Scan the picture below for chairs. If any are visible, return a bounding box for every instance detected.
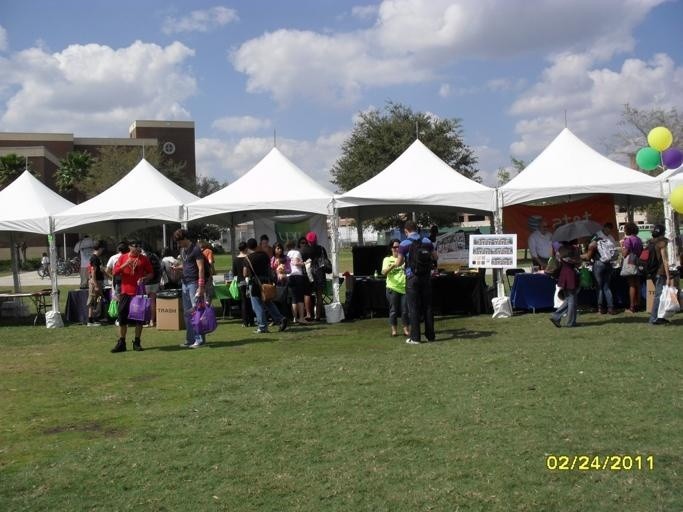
[506,269,525,292]
[39,288,61,313]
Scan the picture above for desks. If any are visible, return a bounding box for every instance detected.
[65,289,116,324]
[213,277,345,320]
[0,292,49,326]
[349,271,489,318]
[509,267,650,314]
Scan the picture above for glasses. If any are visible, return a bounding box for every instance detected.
[391,245,399,249]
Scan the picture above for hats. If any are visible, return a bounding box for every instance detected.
[306,232,317,243]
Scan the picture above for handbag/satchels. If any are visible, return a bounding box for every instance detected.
[666,282,681,311]
[318,247,332,273]
[545,256,563,276]
[246,255,276,302]
[129,283,151,322]
[657,282,675,318]
[620,238,638,276]
[192,293,216,334]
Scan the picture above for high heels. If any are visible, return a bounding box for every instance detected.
[403,328,410,336]
[391,329,397,336]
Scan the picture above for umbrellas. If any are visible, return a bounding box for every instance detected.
[549,219,603,242]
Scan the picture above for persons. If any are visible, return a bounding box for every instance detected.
[301,231,328,321]
[202,245,215,307]
[42,253,51,280]
[243,238,288,334]
[298,237,309,249]
[382,239,409,336]
[86,240,106,326]
[259,233,274,257]
[106,241,125,326]
[549,222,580,327]
[602,223,613,235]
[579,234,618,314]
[648,225,670,325]
[429,225,439,241]
[396,221,438,344]
[528,219,554,270]
[112,237,154,352]
[139,241,162,328]
[169,229,206,347]
[232,240,255,327]
[270,242,292,322]
[621,222,643,313]
[287,239,310,325]
[160,246,180,288]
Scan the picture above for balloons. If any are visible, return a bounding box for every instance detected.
[647,127,673,151]
[661,148,682,169]
[635,148,660,171]
[669,184,683,213]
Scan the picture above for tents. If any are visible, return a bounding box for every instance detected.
[496,126,661,298]
[51,159,201,310]
[184,146,339,303]
[0,170,77,310]
[657,162,683,271]
[333,139,497,302]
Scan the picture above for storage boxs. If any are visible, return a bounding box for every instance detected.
[155,298,183,331]
[646,276,679,313]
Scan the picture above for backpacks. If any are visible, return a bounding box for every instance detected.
[638,236,667,278]
[589,238,618,264]
[406,236,435,276]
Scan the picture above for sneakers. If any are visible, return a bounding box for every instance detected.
[624,307,634,313]
[406,338,421,345]
[254,326,269,333]
[132,341,143,351]
[111,340,127,352]
[189,342,206,348]
[181,343,192,347]
[278,318,287,332]
[87,322,102,327]
[549,316,562,328]
